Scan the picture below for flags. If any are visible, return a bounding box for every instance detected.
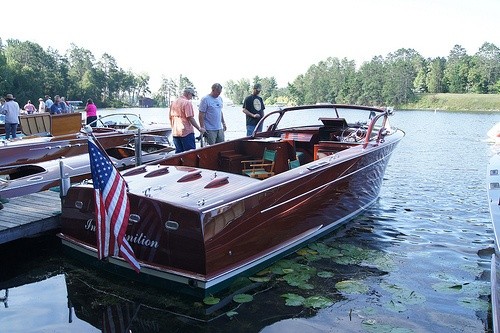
[87,134,141,274]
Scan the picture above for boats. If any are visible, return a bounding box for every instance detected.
[61,197,406,332]
[0,132,177,198]
[55,104,406,298]
[0,113,173,168]
[487,121,500,258]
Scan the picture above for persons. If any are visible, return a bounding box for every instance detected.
[84,99,98,127]
[0,98,6,113]
[38,95,75,115]
[242,83,266,137]
[24,100,37,114]
[198,83,227,146]
[4,94,21,139]
[168,87,208,155]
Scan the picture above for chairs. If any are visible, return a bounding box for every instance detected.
[288,155,300,170]
[241,146,277,178]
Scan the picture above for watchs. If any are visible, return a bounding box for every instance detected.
[222,123,226,125]
[199,128,201,130]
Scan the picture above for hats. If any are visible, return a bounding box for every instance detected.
[184,87,195,96]
[4,94,15,100]
[253,84,262,91]
